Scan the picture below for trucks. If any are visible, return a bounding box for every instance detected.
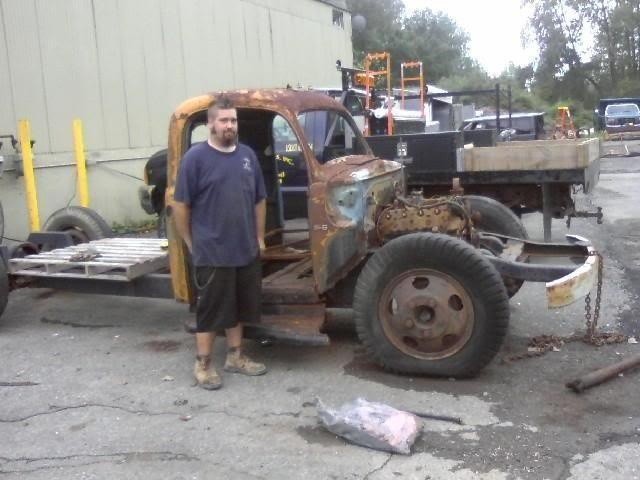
[0,86,606,380]
[594,97,640,133]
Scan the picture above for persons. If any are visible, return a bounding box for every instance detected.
[173,99,270,390]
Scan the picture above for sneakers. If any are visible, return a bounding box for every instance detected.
[194,352,222,390]
[223,350,267,377]
